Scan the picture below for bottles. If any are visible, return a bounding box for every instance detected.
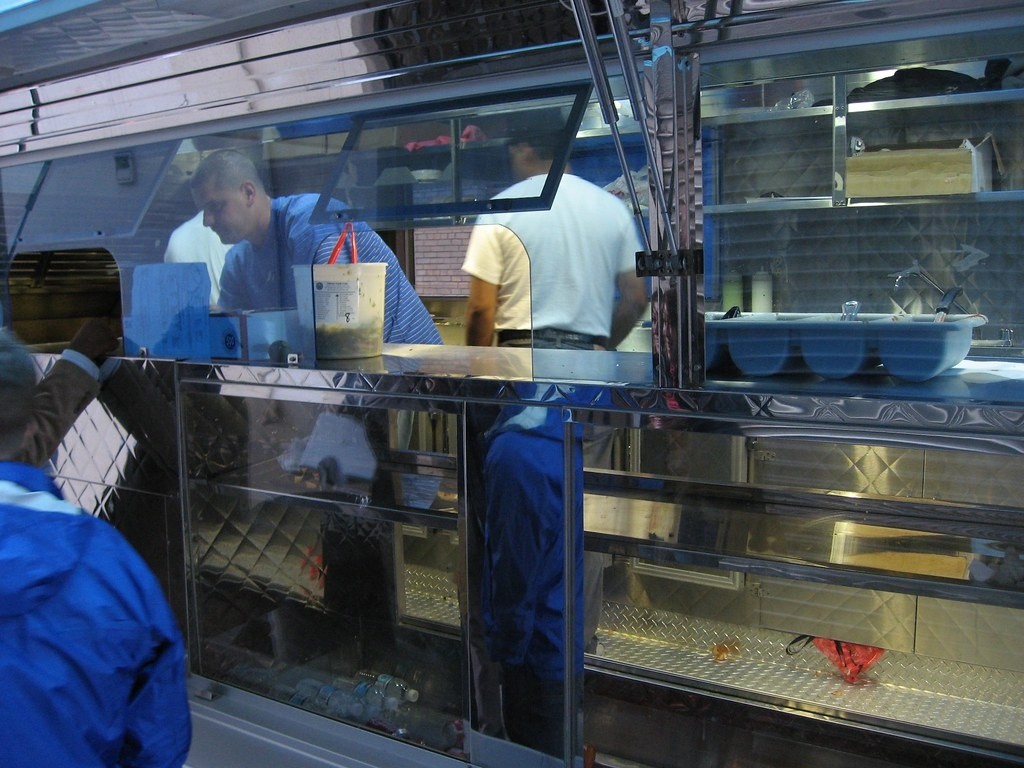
[268,654,435,719]
[722,273,743,312]
[751,266,772,313]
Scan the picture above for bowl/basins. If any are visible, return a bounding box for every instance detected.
[372,165,418,187]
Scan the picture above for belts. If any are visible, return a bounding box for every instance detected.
[498,330,610,351]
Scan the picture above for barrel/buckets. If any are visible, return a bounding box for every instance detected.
[292,222,388,360]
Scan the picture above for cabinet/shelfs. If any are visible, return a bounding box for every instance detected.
[253,28,1024,241]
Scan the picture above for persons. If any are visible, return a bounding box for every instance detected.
[0,316,191,768]
[194,148,449,508]
[479,393,584,758]
[164,208,237,311]
[460,108,651,656]
